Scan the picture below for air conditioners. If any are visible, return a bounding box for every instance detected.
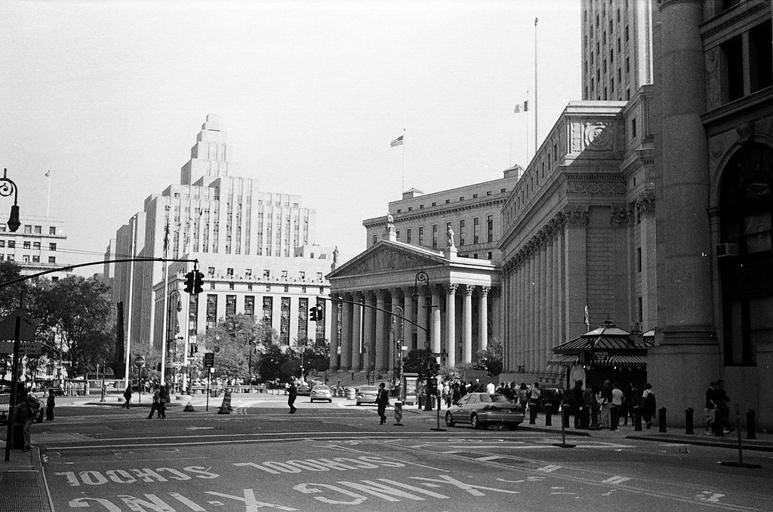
[715,242,738,257]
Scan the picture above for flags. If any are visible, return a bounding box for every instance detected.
[42,169,54,180]
[514,100,529,114]
[582,304,593,327]
[389,135,405,148]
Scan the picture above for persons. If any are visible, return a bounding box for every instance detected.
[372,374,661,428]
[145,384,165,419]
[157,385,166,418]
[716,377,735,433]
[703,381,731,441]
[122,383,135,410]
[12,385,58,453]
[286,382,298,414]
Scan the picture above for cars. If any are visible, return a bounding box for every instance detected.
[0,393,45,423]
[309,384,332,403]
[354,384,378,406]
[443,392,526,431]
[285,381,308,392]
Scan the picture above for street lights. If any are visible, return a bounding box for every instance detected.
[247,333,256,374]
[384,326,395,378]
[410,270,434,411]
[390,304,404,402]
[0,167,21,234]
[165,288,181,402]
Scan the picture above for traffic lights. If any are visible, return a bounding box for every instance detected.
[193,269,205,294]
[315,302,324,321]
[182,270,194,294]
[308,306,316,322]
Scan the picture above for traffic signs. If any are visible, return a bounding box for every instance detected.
[204,352,214,367]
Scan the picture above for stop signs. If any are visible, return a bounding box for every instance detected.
[132,356,144,368]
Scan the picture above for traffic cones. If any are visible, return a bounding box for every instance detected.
[216,389,233,415]
[182,401,195,412]
